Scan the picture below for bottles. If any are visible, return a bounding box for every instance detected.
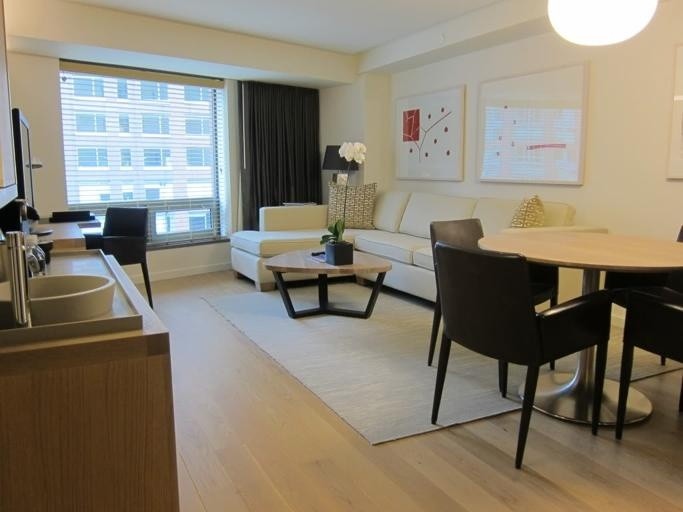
[23,233,47,277]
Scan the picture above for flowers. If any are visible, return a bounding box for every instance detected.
[319,142,368,243]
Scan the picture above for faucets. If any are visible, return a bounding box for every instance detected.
[5,230,46,328]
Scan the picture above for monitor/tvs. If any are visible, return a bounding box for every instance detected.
[11,107,33,213]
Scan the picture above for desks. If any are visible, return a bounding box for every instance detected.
[478,226,683,426]
[28,222,87,250]
[38,216,101,228]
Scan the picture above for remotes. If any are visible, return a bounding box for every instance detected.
[312,252,324,256]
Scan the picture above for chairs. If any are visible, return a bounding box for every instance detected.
[430,218,559,392]
[614,286,682,442]
[431,241,608,468]
[601,225,683,365]
[83,207,153,310]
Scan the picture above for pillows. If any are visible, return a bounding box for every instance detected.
[510,194,551,228]
[327,181,379,231]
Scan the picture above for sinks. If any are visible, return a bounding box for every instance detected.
[0,274,117,324]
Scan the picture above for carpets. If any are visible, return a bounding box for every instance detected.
[200,279,682,445]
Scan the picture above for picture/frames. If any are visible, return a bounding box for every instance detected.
[476,62,587,186]
[667,46,683,180]
[394,84,464,182]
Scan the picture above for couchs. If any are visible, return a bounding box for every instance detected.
[231,193,611,312]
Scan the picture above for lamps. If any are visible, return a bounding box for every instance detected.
[547,1,659,46]
[24,155,43,169]
[322,145,359,183]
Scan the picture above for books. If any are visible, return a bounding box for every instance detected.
[309,253,326,264]
